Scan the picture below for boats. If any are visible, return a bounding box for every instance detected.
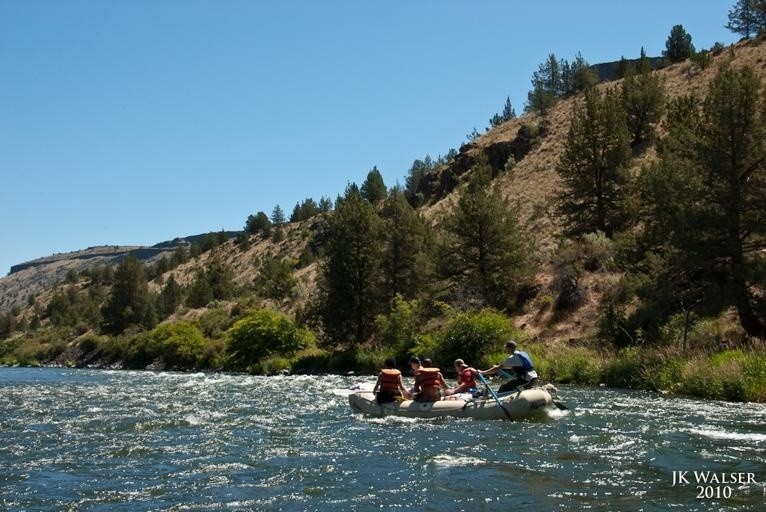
[349,387,553,418]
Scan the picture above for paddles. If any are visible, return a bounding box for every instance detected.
[478,373,513,422]
[393,395,406,400]
[493,365,570,410]
[333,389,373,396]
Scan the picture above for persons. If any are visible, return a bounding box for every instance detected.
[476,340,539,396]
[443,359,478,395]
[405,356,425,394]
[413,358,450,402]
[372,357,408,404]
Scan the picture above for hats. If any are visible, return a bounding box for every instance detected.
[454,359,468,368]
[503,340,517,348]
